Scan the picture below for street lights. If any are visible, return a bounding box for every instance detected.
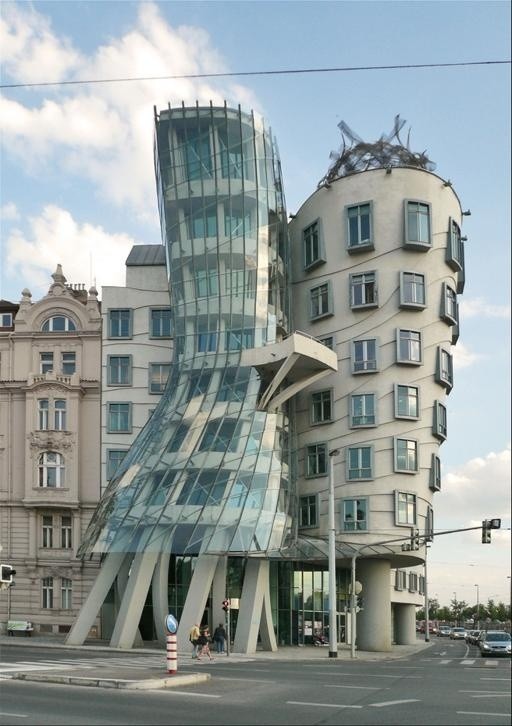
[452,590,459,627]
[326,444,344,657]
[473,583,480,630]
[506,575,512,579]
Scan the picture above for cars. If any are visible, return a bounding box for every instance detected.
[479,629,512,658]
[415,618,511,646]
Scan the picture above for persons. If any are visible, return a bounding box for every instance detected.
[189,623,201,659]
[195,625,214,661]
[211,623,227,654]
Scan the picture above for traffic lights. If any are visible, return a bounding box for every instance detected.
[355,593,362,613]
[221,599,229,612]
[1,563,17,583]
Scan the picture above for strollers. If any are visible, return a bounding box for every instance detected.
[314,634,329,646]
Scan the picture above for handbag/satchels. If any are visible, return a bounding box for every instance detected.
[196,635,207,645]
